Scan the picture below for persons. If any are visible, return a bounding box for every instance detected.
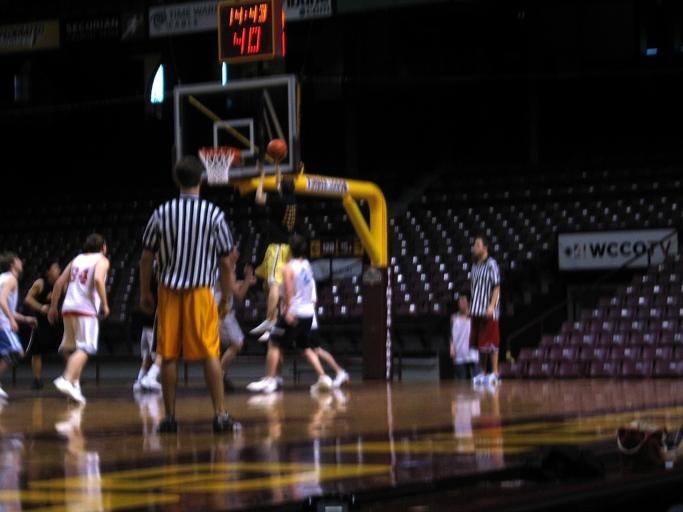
[45,232,111,398]
[136,156,235,433]
[449,292,478,380]
[248,159,297,343]
[0,249,39,402]
[131,234,351,394]
[21,258,65,391]
[469,235,501,385]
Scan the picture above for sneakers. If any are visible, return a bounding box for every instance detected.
[246,376,283,392]
[133,377,162,392]
[310,370,350,390]
[53,376,86,402]
[249,319,271,341]
[161,417,177,432]
[471,373,501,384]
[214,414,242,432]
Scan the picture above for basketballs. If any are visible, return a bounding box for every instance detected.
[267,138,288,161]
